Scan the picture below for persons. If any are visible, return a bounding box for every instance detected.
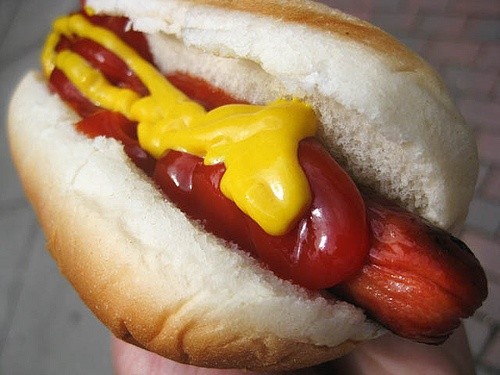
[115,317,500,375]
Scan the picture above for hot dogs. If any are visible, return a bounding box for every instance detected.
[7,1,488,371]
